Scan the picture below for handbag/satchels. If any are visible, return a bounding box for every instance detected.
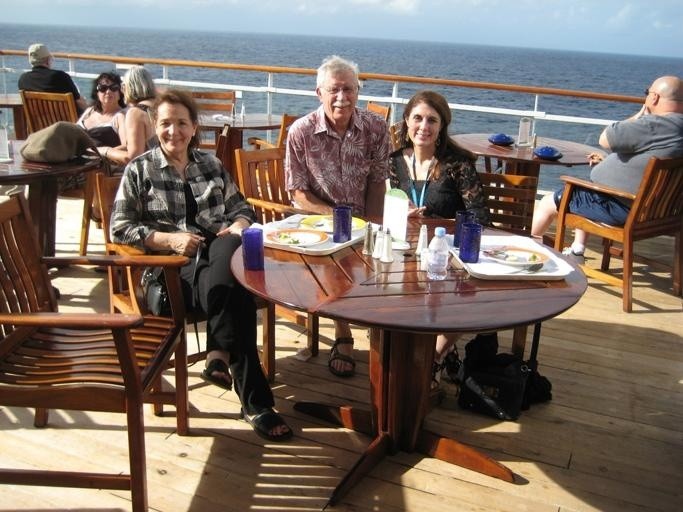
[146,280,171,316]
[20,121,96,162]
[457,364,531,422]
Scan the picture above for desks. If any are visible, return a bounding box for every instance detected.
[196,114,285,176]
[1,138,105,269]
[450,133,608,237]
[229,215,586,508]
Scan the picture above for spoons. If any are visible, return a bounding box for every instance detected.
[510,263,544,274]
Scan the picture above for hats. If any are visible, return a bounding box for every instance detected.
[29,44,51,60]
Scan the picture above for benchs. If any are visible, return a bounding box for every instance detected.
[110,91,235,148]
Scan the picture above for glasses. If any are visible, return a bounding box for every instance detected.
[643,91,657,95]
[96,83,120,92]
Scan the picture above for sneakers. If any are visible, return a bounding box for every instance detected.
[562,246,584,265]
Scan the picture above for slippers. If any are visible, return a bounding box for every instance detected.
[244,410,293,441]
[200,359,232,391]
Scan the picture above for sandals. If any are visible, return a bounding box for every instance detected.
[431,344,461,389]
[328,337,355,377]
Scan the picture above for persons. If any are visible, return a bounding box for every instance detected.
[531,75,683,265]
[284,53,390,378]
[81,66,162,166]
[18,43,88,119]
[110,86,293,441]
[57,72,127,193]
[389,91,492,397]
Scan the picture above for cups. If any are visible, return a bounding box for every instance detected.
[240,226,265,271]
[332,206,353,243]
[457,221,484,264]
[453,209,476,248]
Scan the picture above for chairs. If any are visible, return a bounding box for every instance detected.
[248,115,304,188]
[368,102,390,123]
[234,148,319,358]
[20,90,78,134]
[0,192,191,511]
[93,166,274,417]
[478,174,538,359]
[214,125,228,156]
[553,156,683,314]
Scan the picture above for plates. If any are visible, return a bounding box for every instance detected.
[301,214,367,233]
[488,133,514,145]
[266,229,330,246]
[533,146,564,160]
[479,246,553,267]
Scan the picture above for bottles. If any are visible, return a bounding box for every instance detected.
[232,120,235,127]
[231,102,236,119]
[380,228,395,263]
[424,281,446,324]
[240,101,246,118]
[371,258,383,290]
[362,220,375,256]
[414,225,431,257]
[362,254,373,280]
[241,118,245,127]
[531,132,538,150]
[420,247,430,271]
[415,256,429,290]
[379,261,392,290]
[426,225,450,280]
[372,225,384,259]
